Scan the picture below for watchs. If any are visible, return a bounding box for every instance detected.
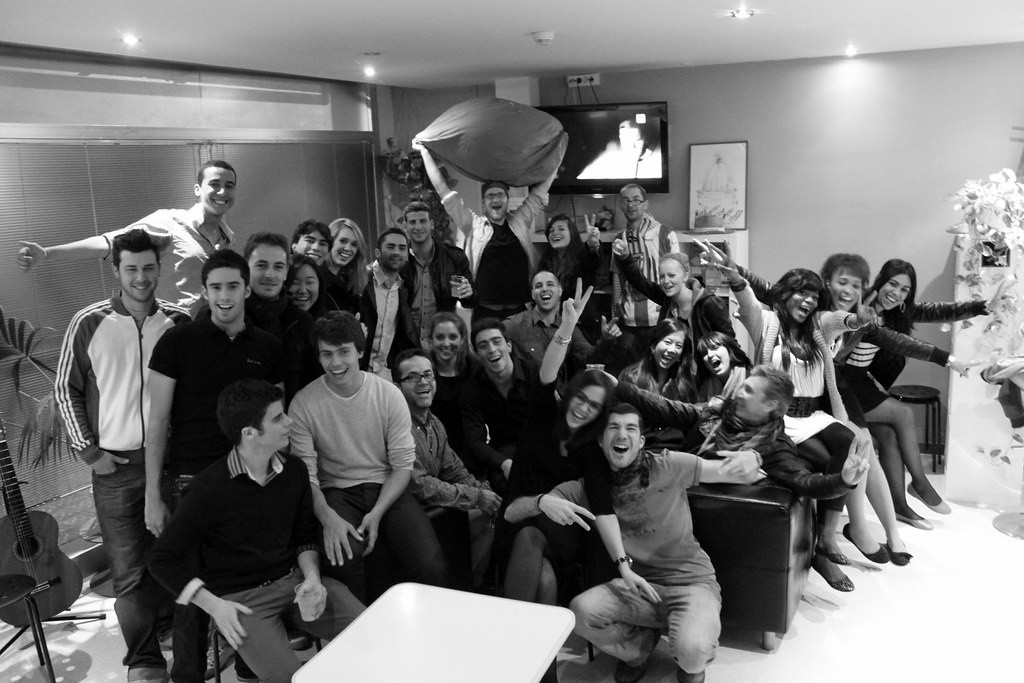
[616,555,632,567]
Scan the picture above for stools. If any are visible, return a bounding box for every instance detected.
[889,385,942,473]
[213,614,322,683]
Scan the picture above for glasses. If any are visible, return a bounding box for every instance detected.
[571,390,600,416]
[622,199,644,206]
[398,372,435,385]
[484,192,506,198]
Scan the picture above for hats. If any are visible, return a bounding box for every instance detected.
[664,252,690,272]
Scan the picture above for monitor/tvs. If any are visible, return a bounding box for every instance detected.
[533,101,670,194]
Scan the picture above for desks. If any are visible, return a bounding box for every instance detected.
[532,229,749,291]
[291,582,576,683]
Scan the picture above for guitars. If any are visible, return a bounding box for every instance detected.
[0,418,85,627]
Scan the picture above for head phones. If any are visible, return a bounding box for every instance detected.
[721,399,750,433]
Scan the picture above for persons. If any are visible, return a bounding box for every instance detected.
[16,132,1015,683]
[619,121,651,161]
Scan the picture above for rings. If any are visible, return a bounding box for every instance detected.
[493,508,497,511]
[146,527,150,529]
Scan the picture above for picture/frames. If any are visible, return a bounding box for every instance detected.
[687,140,749,230]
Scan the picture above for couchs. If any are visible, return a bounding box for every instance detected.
[686,451,824,650]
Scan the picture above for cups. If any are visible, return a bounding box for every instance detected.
[586,364,605,372]
[294,583,321,621]
[452,275,463,298]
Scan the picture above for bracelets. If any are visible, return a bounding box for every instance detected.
[587,241,600,257]
[946,355,956,366]
[537,495,544,508]
[554,329,571,345]
[721,277,742,284]
[847,315,860,331]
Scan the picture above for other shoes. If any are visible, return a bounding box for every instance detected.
[811,552,856,592]
[678,664,705,683]
[290,637,310,651]
[235,667,258,681]
[843,524,891,564]
[203,644,234,680]
[895,507,932,530]
[616,628,662,683]
[815,542,850,565]
[886,539,911,566]
[128,665,170,683]
[908,481,951,514]
